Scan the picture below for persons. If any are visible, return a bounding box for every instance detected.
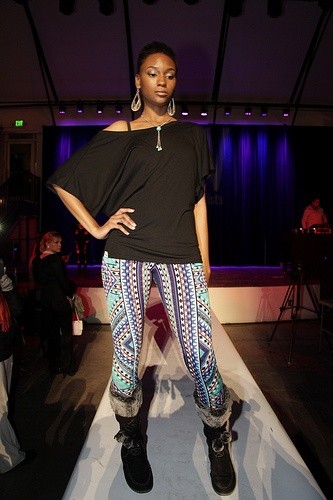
[0,231,78,472]
[300,196,330,229]
[47,41,244,496]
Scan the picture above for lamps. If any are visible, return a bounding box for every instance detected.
[182,102,290,118]
[58,0,333,18]
[58,103,121,114]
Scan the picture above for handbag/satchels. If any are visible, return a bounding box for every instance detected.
[72,306,83,336]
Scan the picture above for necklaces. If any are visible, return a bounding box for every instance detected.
[142,115,173,151]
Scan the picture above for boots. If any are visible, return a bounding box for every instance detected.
[192,384,237,495]
[107,383,155,494]
